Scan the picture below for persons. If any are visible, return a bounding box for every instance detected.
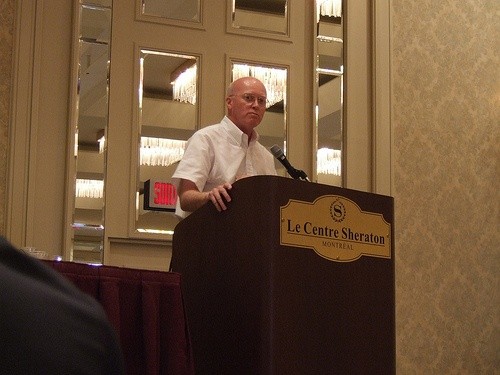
[0,234,123,375]
[174,77,279,222]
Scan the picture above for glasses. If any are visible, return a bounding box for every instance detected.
[228,94,269,104]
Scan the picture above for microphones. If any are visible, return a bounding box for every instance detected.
[270,144,300,181]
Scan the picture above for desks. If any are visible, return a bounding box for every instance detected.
[38,259,196,375]
